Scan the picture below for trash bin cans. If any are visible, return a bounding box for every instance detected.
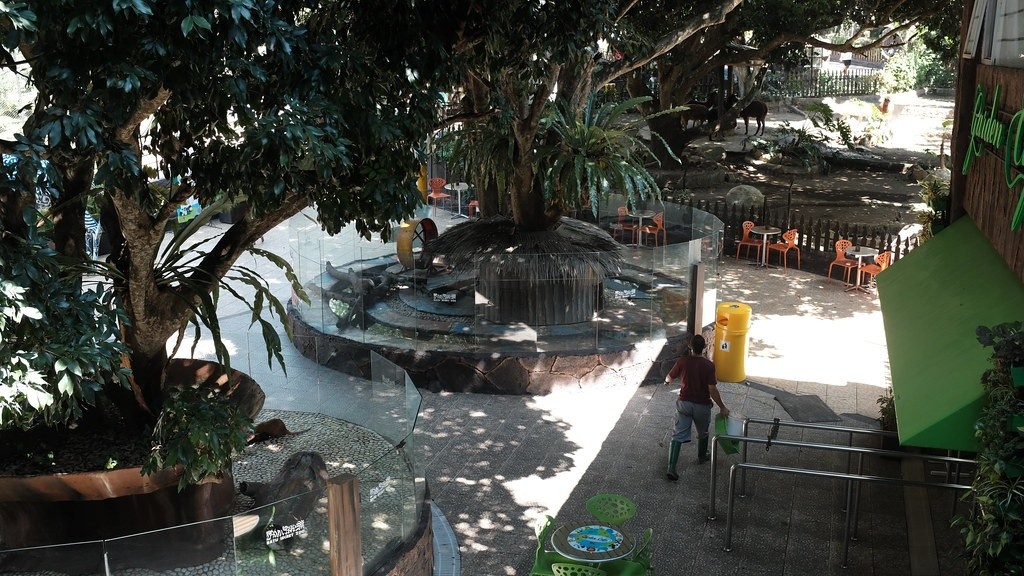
[715,301,752,384]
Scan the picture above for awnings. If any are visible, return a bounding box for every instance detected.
[875,214,1024,451]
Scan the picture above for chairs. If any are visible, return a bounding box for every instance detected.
[426,178,453,217]
[766,229,800,274]
[828,240,866,288]
[641,211,668,250]
[613,207,639,244]
[469,201,479,217]
[736,221,770,267]
[528,493,653,576]
[856,251,892,299]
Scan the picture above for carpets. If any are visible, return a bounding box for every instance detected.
[776,394,843,423]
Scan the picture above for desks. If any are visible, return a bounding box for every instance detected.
[551,520,637,568]
[844,246,880,294]
[444,182,474,219]
[626,210,656,249]
[746,226,781,270]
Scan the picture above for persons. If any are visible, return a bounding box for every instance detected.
[840,52,852,73]
[665,334,729,480]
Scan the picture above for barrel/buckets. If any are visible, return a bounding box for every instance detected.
[727,411,746,436]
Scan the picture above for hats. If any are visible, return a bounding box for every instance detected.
[687,334,708,348]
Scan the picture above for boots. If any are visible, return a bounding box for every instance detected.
[697,433,711,463]
[666,440,682,481]
